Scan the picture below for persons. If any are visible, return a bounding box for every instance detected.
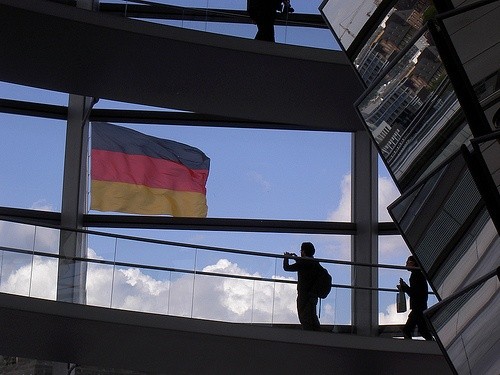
[282,241,332,328]
[397,255,435,341]
[247,0,295,43]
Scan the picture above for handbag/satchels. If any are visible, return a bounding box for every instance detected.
[396,280,407,313]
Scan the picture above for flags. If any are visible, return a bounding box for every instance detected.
[90,121,210,216]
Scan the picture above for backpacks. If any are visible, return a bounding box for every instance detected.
[312,264,332,299]
